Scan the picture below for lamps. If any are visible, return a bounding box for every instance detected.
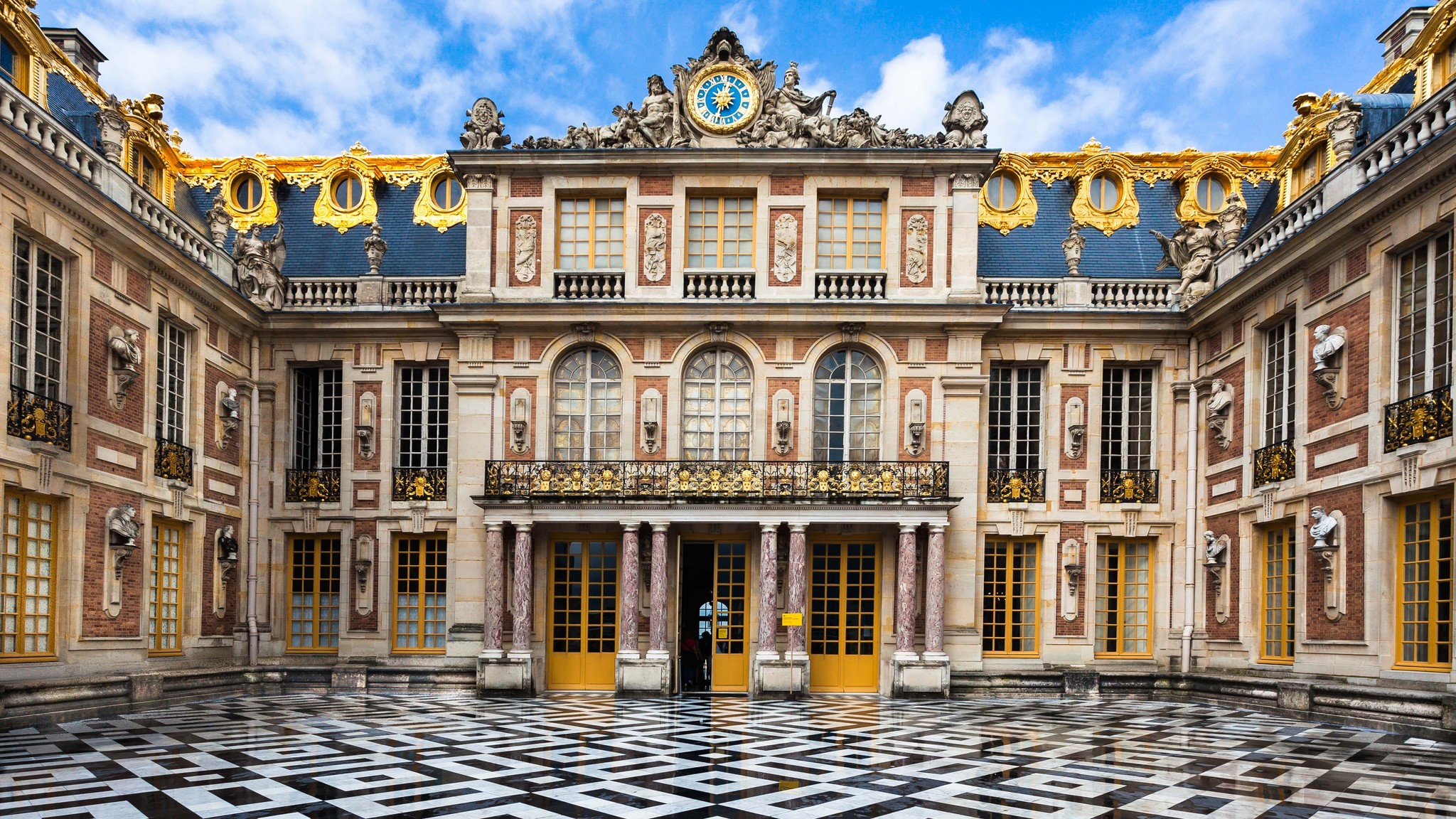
[509,389,534,454]
[640,391,661,455]
[1063,542,1083,598]
[905,394,926,457]
[1065,401,1085,459]
[770,392,795,456]
[353,535,373,595]
[354,393,378,459]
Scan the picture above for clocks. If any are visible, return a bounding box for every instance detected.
[684,64,763,134]
